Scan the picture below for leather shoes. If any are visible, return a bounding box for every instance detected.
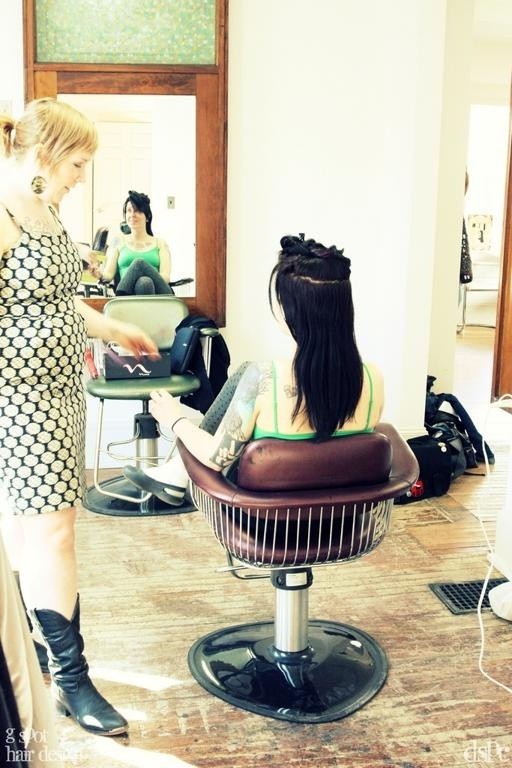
[122,464,187,507]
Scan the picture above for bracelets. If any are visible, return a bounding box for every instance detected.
[171,416,188,431]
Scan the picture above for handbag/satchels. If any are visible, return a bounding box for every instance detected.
[393,435,452,504]
[428,423,467,480]
[426,372,494,467]
[170,326,199,375]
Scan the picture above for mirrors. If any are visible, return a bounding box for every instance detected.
[22,66,230,334]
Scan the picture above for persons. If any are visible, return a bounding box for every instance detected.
[4,96,161,740]
[88,188,175,297]
[124,230,387,550]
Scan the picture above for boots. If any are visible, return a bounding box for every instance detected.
[12,571,49,672]
[25,593,129,737]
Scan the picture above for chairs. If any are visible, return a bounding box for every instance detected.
[81,296,423,725]
